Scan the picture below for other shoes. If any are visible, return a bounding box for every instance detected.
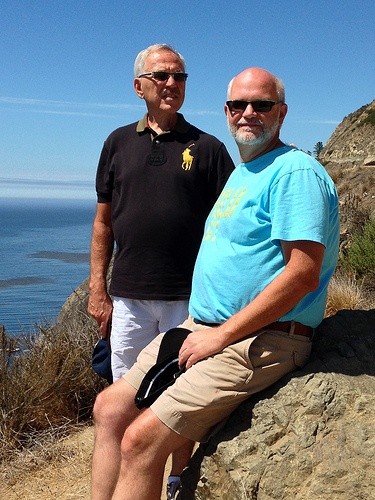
[166,475,181,500]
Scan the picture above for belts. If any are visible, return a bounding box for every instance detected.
[193,317,313,340]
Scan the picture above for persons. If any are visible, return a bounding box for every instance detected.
[91,66,341,500]
[86,43,236,500]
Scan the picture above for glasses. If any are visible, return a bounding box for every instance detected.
[138,72,188,82]
[226,100,283,112]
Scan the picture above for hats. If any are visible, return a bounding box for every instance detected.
[91,319,113,385]
[134,328,196,409]
